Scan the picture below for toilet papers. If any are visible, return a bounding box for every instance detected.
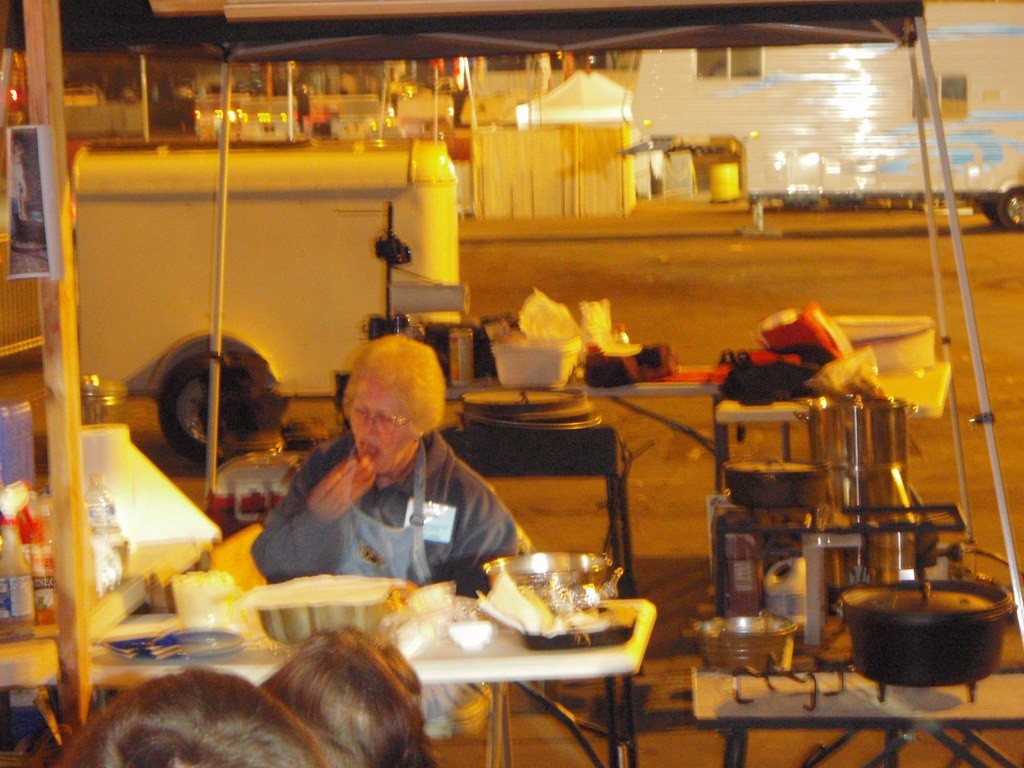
[81,423,223,585]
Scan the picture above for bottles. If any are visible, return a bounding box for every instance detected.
[0,519,36,642]
[723,510,757,619]
[763,558,807,625]
[615,321,630,342]
[30,506,54,625]
[84,474,116,536]
[450,327,475,387]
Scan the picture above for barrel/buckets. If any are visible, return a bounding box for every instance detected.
[713,163,738,198]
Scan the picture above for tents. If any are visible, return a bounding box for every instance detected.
[0,0,1024,732]
[515,69,633,125]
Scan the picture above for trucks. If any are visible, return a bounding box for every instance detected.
[2,138,464,468]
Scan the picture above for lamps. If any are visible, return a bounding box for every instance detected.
[514,51,632,130]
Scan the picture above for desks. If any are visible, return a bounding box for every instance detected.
[691,666,1024,768]
[444,366,953,616]
[0,598,658,768]
[0,538,212,753]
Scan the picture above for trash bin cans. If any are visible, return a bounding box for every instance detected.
[710,162,740,202]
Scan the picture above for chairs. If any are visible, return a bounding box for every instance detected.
[443,409,639,768]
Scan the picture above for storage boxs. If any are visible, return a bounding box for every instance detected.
[834,312,936,373]
[490,339,578,388]
[472,125,637,222]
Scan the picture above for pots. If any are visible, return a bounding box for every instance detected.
[722,456,832,506]
[840,579,1014,687]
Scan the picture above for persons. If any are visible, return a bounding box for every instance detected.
[260,628,436,768]
[250,334,518,586]
[52,667,327,768]
[11,139,28,236]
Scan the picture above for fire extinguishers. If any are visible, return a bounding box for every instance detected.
[705,490,762,618]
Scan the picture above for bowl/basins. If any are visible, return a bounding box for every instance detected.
[483,551,614,614]
[698,612,799,673]
[450,621,490,650]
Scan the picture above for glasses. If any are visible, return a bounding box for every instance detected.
[344,404,410,433]
